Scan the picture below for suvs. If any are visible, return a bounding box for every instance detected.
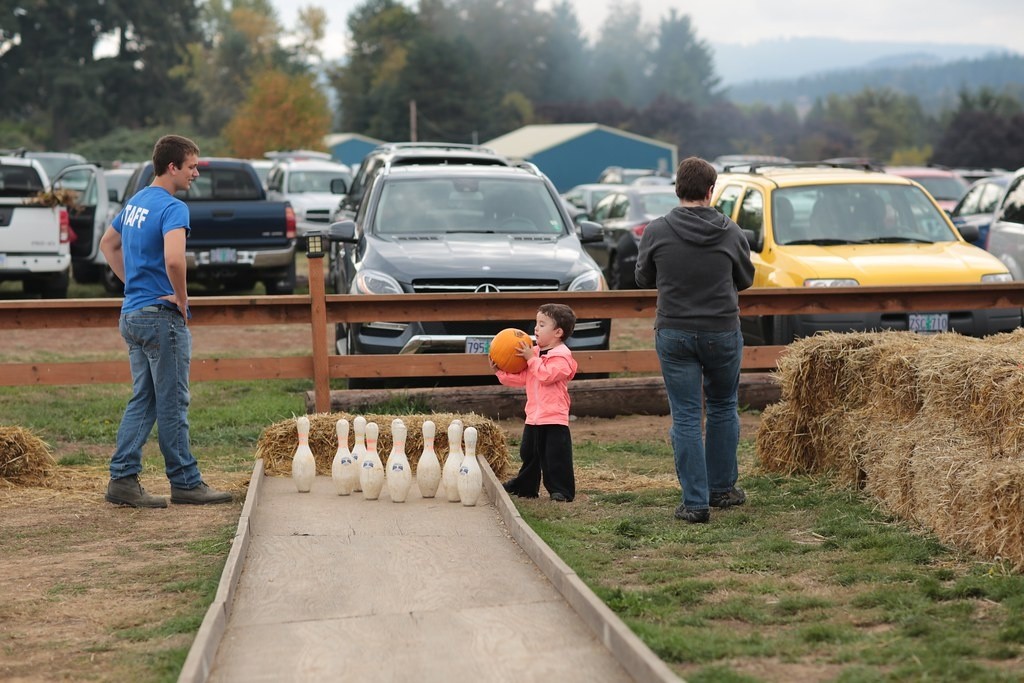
[326,161,611,390]
[329,141,508,289]
[709,162,1022,344]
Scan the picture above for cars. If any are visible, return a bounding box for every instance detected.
[599,167,660,189]
[1,151,91,190]
[263,160,354,237]
[74,170,136,284]
[986,167,1024,283]
[559,185,630,229]
[710,165,817,229]
[877,167,971,236]
[932,177,1011,252]
[577,189,680,290]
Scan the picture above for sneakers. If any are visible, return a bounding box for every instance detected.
[170,480,233,504]
[105,473,167,508]
[674,504,710,523]
[709,488,746,508]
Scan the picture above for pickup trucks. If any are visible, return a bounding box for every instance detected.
[102,157,296,297]
[0,157,111,301]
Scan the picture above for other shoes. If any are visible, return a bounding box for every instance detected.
[551,493,568,501]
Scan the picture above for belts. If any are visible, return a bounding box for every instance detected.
[151,304,173,312]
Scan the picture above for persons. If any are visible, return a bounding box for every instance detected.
[635,157,755,522]
[100,136,232,508]
[488,304,578,503]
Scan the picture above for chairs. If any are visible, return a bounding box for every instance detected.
[484,191,530,230]
[774,195,809,241]
[388,192,440,228]
[809,193,885,238]
